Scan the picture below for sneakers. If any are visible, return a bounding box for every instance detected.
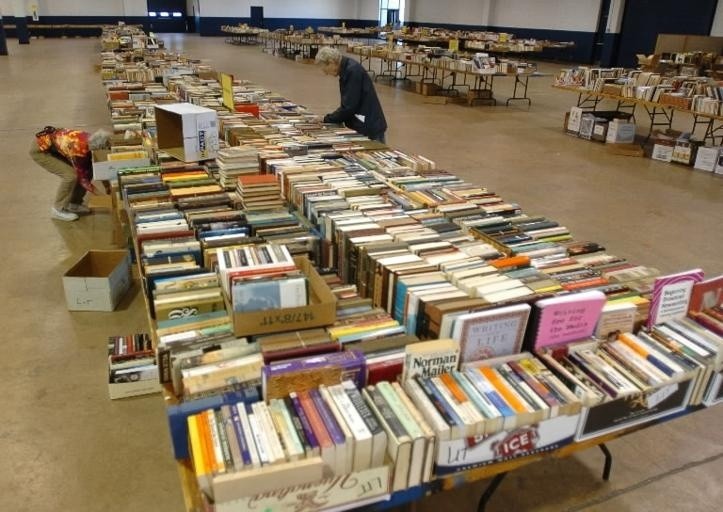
[49,206,79,222]
[66,202,90,213]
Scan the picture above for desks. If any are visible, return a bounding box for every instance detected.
[128,216,704,512]
[225,32,259,44]
[261,36,347,60]
[346,51,533,106]
[551,84,723,149]
[323,31,371,45]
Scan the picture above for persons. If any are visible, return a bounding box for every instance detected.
[28,127,113,221]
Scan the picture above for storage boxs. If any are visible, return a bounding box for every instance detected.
[416,81,437,96]
[62,250,133,312]
[154,103,219,163]
[468,89,490,105]
[108,357,162,400]
[92,149,150,181]
[564,107,723,175]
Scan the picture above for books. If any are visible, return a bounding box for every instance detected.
[98,21,722,512]
